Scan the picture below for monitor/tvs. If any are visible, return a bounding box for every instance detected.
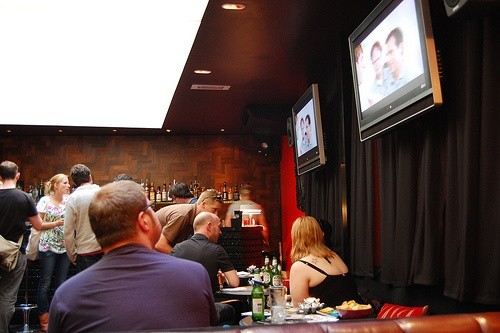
[348,0,444,142]
[291,83,326,176]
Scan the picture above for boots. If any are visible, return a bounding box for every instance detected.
[39,313,49,331]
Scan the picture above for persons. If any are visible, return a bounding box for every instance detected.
[169,182,198,205]
[227,181,271,253]
[153,187,224,258]
[48,180,221,333]
[291,112,316,157]
[63,163,104,273]
[315,219,344,264]
[354,27,422,109]
[35,173,73,333]
[0,161,65,333]
[171,210,240,327]
[288,216,362,308]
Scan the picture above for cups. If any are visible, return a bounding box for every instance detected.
[217,272,224,290]
[269,286,287,325]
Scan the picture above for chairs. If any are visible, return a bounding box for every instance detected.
[376,303,429,320]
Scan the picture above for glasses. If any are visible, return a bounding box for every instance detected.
[202,195,223,202]
[141,201,156,213]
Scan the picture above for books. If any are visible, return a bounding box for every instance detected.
[221,287,247,292]
[241,306,340,325]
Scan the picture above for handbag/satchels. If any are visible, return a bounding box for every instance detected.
[0,235,22,269]
[26,197,47,260]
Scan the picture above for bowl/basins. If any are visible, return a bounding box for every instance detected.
[336,304,373,319]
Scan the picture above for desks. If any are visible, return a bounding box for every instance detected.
[217,271,265,301]
[239,317,374,327]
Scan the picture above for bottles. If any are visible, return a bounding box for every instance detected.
[71,184,77,193]
[24,322,29,333]
[251,255,283,322]
[16,180,45,201]
[140,177,240,202]
